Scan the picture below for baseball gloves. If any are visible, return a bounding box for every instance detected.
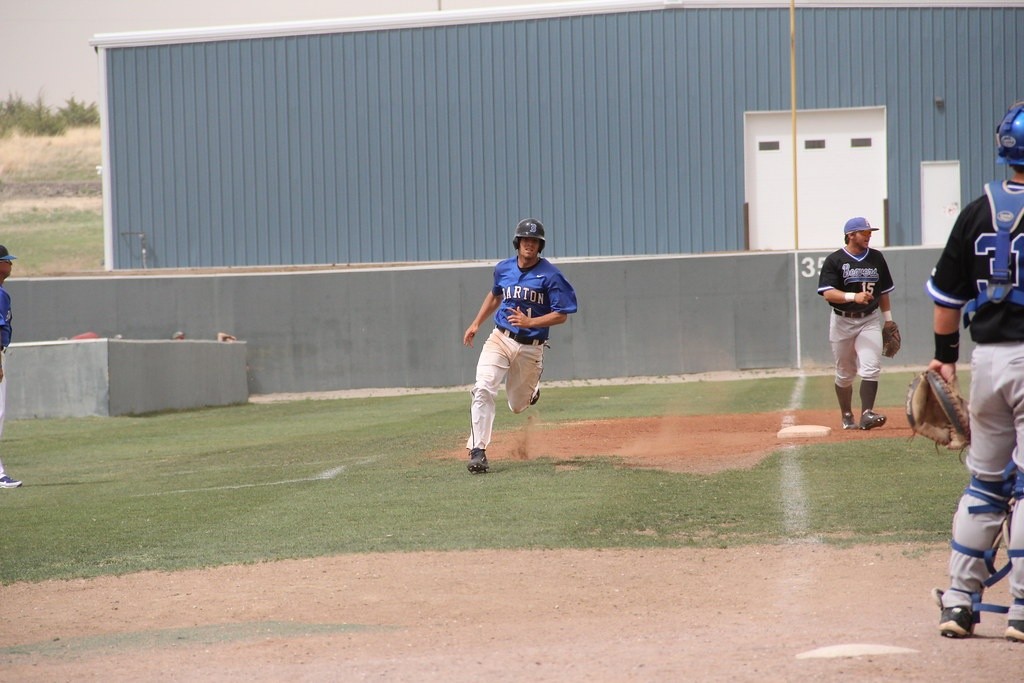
[882,320,902,358]
[905,371,973,453]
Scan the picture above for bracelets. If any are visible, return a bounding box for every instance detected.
[934,329,960,363]
[0,365,2,368]
[845,293,856,301]
[883,311,892,321]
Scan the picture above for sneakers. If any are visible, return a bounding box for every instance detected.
[1004,619,1024,642]
[467,448,490,473]
[841,412,858,429]
[858,410,887,429]
[939,605,977,638]
[531,388,540,405]
[1,474,23,489]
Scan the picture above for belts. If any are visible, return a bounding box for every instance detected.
[497,324,546,346]
[833,304,880,318]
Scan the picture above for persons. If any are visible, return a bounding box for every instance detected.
[818,218,902,430]
[464,219,578,472]
[0,244,23,488]
[925,101,1024,641]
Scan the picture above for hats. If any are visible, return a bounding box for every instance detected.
[0,243,17,260]
[844,217,880,233]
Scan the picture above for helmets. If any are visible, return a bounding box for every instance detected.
[514,218,545,253]
[996,102,1024,166]
